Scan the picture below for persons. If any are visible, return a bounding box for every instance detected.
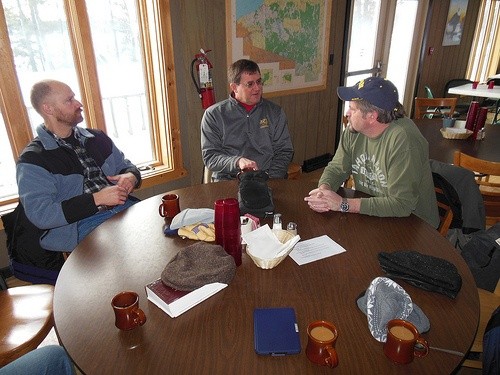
[303,76,440,231]
[200,59,295,183]
[0,345,77,375]
[15,80,142,253]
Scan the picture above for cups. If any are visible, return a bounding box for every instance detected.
[159,193,181,220]
[215,198,242,267]
[383,320,429,364]
[443,117,456,128]
[111,291,146,331]
[488,81,494,89]
[240,215,257,244]
[454,120,466,129]
[465,100,488,140]
[472,80,479,89]
[305,321,339,369]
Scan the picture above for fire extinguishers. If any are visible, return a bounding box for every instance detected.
[196,48,216,109]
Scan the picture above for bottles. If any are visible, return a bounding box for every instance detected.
[272,214,283,229]
[287,221,297,235]
[263,211,274,229]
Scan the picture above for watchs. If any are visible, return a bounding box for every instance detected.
[341,198,349,214]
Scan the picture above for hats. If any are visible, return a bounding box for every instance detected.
[336,76,398,112]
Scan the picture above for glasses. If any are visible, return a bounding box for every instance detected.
[236,79,263,87]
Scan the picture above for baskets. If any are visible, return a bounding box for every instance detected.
[245,228,296,270]
[440,126,474,140]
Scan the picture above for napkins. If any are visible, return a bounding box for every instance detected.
[243,223,301,259]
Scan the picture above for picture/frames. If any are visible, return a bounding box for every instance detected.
[442,0,469,46]
[225,0,332,99]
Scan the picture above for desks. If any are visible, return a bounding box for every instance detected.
[413,120,500,165]
[53,179,480,374]
[448,83,500,99]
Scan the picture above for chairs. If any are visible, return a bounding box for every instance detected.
[0,198,69,368]
[341,78,500,375]
